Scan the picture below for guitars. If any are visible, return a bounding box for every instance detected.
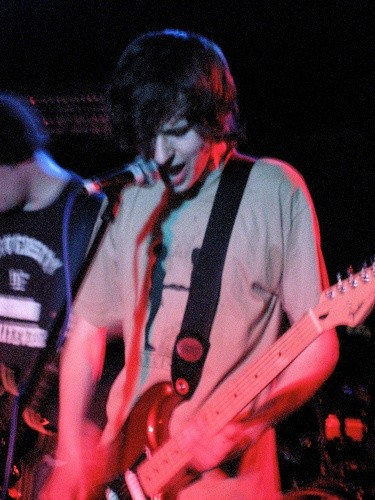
[35,262,375,500]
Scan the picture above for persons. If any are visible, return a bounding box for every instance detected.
[34,27,340,500]
[0,92,128,500]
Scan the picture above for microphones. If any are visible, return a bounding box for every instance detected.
[75,158,159,197]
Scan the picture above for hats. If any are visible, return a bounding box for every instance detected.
[0,93,47,165]
